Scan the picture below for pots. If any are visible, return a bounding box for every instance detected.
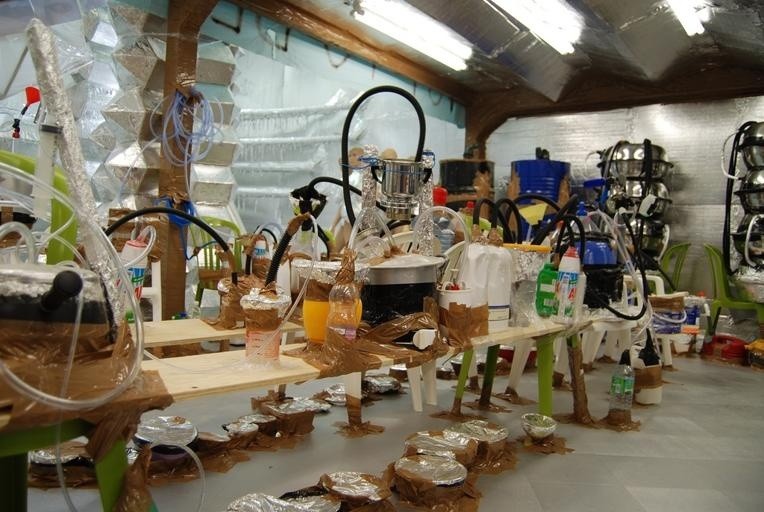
[0,264,109,325]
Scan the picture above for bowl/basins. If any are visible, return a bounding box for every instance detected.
[521,412,557,440]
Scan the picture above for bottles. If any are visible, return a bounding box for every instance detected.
[117,239,149,325]
[606,348,636,425]
[454,200,473,243]
[251,240,267,275]
[551,244,580,326]
[323,280,359,346]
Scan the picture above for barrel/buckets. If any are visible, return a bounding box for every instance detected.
[439,157,495,213]
[511,158,570,242]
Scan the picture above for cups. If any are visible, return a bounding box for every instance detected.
[238,293,292,369]
